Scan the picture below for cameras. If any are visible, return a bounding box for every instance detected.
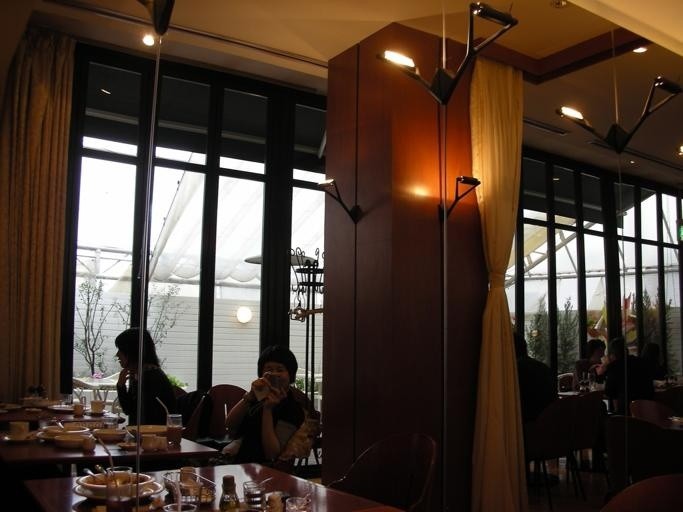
[270,372,289,388]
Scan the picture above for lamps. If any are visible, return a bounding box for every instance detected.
[373,1,523,108]
[556,77,683,150]
[316,177,365,226]
[438,174,482,218]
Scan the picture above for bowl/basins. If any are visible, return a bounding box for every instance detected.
[126,425,186,438]
[93,429,125,440]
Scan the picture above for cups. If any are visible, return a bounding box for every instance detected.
[242,481,312,512]
[89,400,105,413]
[73,403,86,416]
[104,472,132,512]
[140,433,166,451]
[82,436,96,451]
[9,422,29,439]
[162,502,197,512]
[101,418,118,430]
[180,467,196,484]
[167,414,182,446]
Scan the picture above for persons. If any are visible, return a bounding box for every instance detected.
[114,326,176,424]
[513,331,559,423]
[588,336,657,471]
[221,344,305,465]
[573,339,608,417]
[639,343,666,378]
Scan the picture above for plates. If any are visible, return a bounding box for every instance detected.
[1,435,35,443]
[47,404,90,412]
[74,471,164,503]
[117,443,137,450]
[70,498,155,512]
[34,425,92,447]
[86,410,110,415]
[25,408,42,413]
[1,404,21,410]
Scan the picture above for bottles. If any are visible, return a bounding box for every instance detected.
[218,474,241,512]
[579,372,598,393]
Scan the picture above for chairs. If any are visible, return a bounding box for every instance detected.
[331,432,441,511]
[516,380,682,510]
[186,384,248,454]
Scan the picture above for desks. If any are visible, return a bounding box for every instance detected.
[1,407,405,511]
